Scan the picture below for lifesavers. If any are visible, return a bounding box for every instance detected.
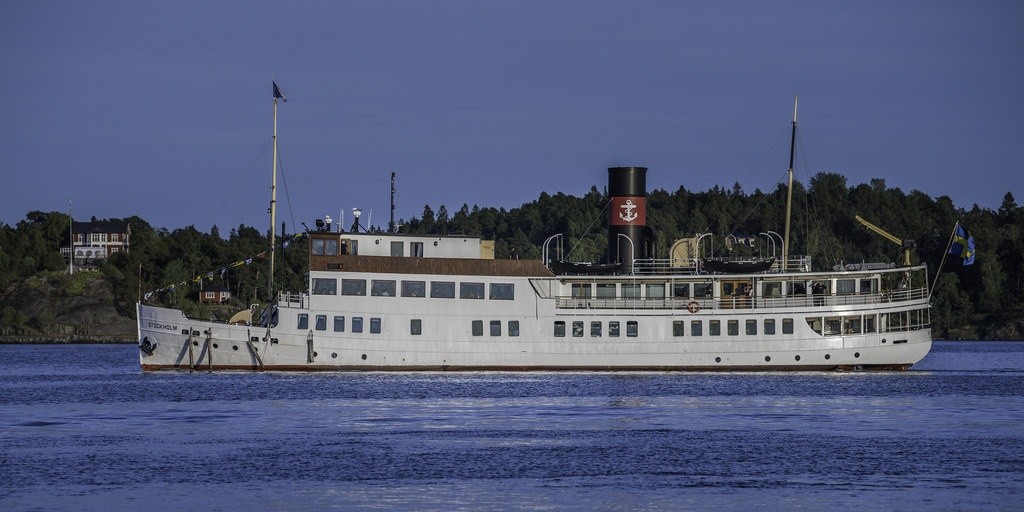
[687,301,700,314]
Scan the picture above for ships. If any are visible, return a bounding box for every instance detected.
[136,79,977,378]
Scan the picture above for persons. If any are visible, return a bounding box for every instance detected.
[812,282,828,305]
[897,275,908,301]
[317,286,496,299]
[705,284,752,308]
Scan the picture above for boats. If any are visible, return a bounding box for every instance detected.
[703,255,775,273]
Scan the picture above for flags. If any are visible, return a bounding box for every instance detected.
[272,80,288,103]
[949,223,974,265]
